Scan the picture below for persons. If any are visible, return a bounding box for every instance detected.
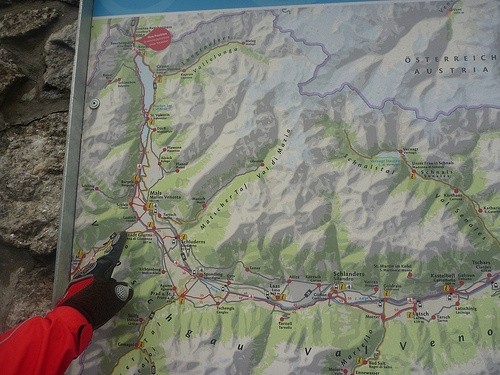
[0,230,134,375]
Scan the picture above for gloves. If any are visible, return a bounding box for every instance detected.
[53,229,134,329]
